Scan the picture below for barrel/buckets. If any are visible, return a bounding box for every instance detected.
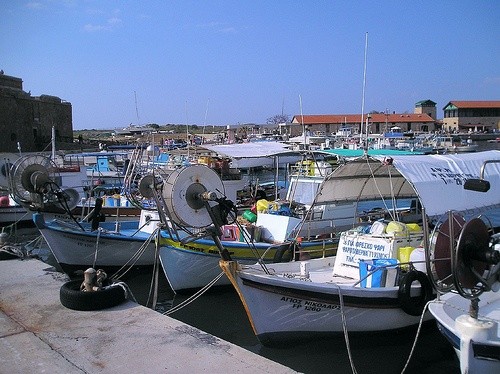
[244,226,261,243]
[241,210,257,222]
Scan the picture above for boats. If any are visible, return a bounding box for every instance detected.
[0,32,500,374]
